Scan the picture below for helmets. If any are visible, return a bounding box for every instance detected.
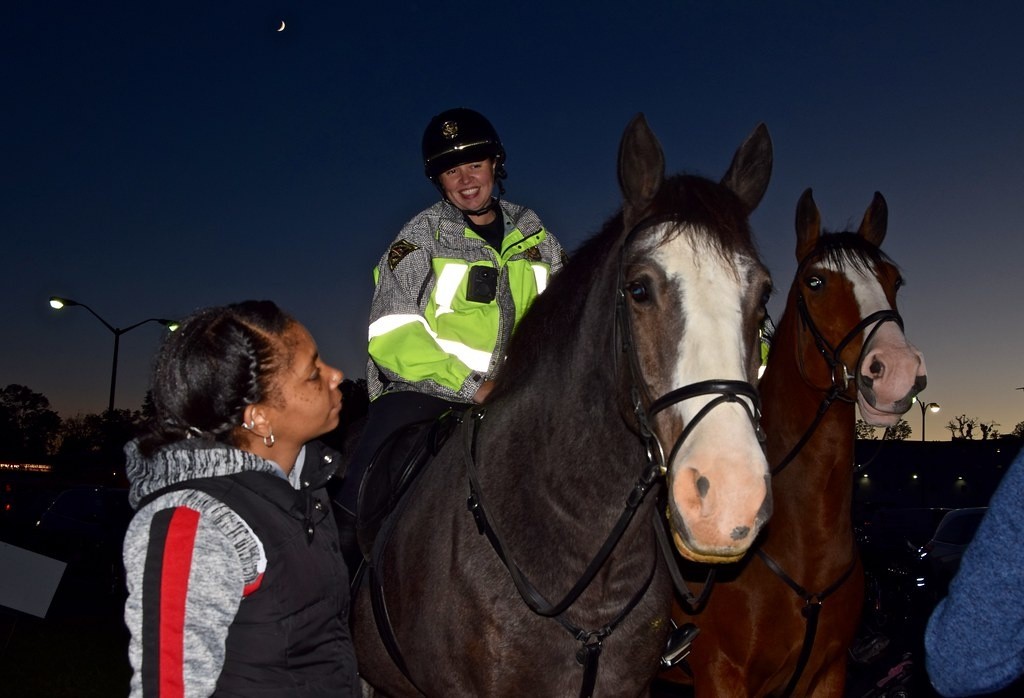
[422,108,506,182]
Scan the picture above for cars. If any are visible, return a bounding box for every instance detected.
[915,506,988,574]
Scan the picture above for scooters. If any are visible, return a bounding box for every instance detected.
[843,630,916,698]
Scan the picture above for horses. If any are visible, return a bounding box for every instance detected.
[328,114,777,698]
[655,187,928,698]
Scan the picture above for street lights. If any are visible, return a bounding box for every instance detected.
[912,394,939,440]
[49,295,180,422]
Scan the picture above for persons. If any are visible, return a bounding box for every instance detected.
[122,299,363,698]
[755,266,777,381]
[923,450,1024,697]
[336,108,564,564]
